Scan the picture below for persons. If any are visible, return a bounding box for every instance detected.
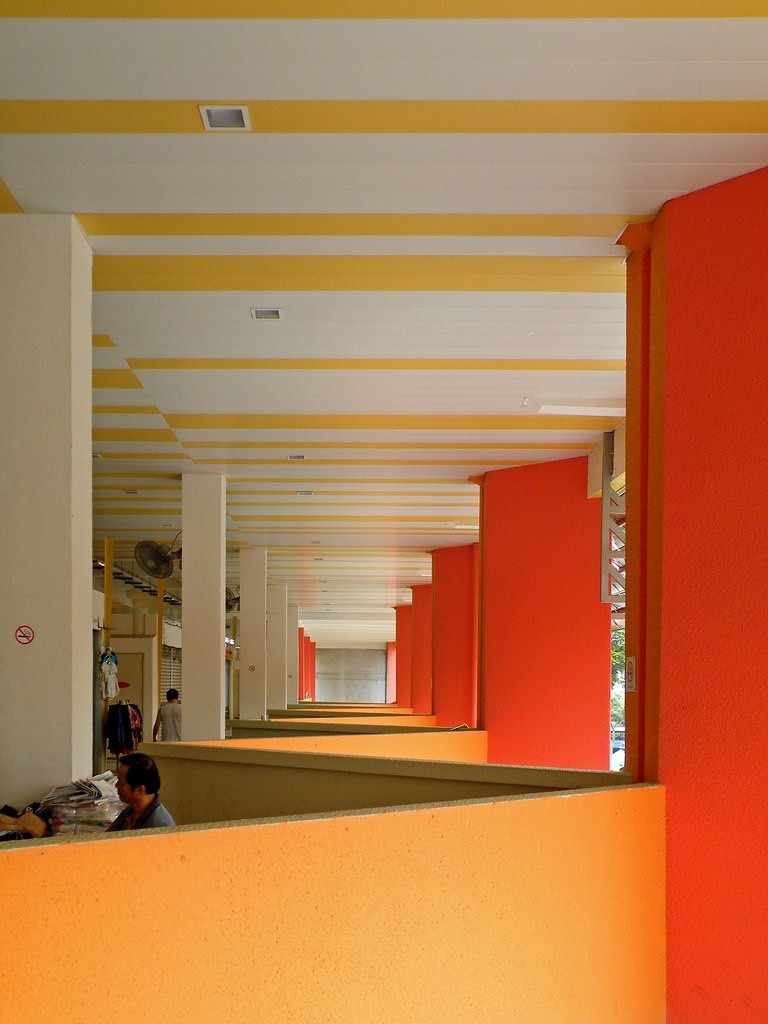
[102,752,177,832]
[152,687,183,743]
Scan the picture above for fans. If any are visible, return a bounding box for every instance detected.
[135,540,182,580]
[225,585,240,613]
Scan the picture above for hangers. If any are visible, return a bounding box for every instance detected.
[97,642,132,711]
